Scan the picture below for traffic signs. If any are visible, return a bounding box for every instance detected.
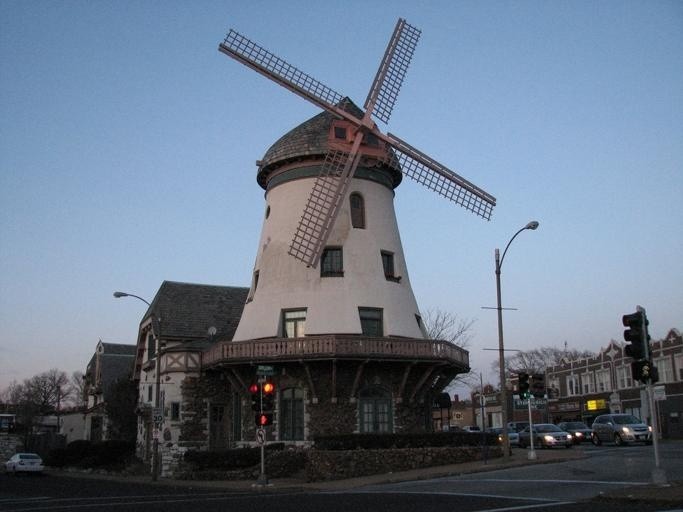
[152,408,164,423]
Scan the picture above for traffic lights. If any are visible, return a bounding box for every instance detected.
[532,374,546,400]
[263,381,275,411]
[255,414,274,427]
[629,361,653,383]
[621,311,647,362]
[250,380,262,413]
[518,371,531,402]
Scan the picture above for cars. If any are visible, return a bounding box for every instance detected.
[440,410,656,450]
[4,453,46,478]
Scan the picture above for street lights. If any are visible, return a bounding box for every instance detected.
[492,219,541,465]
[111,290,166,489]
